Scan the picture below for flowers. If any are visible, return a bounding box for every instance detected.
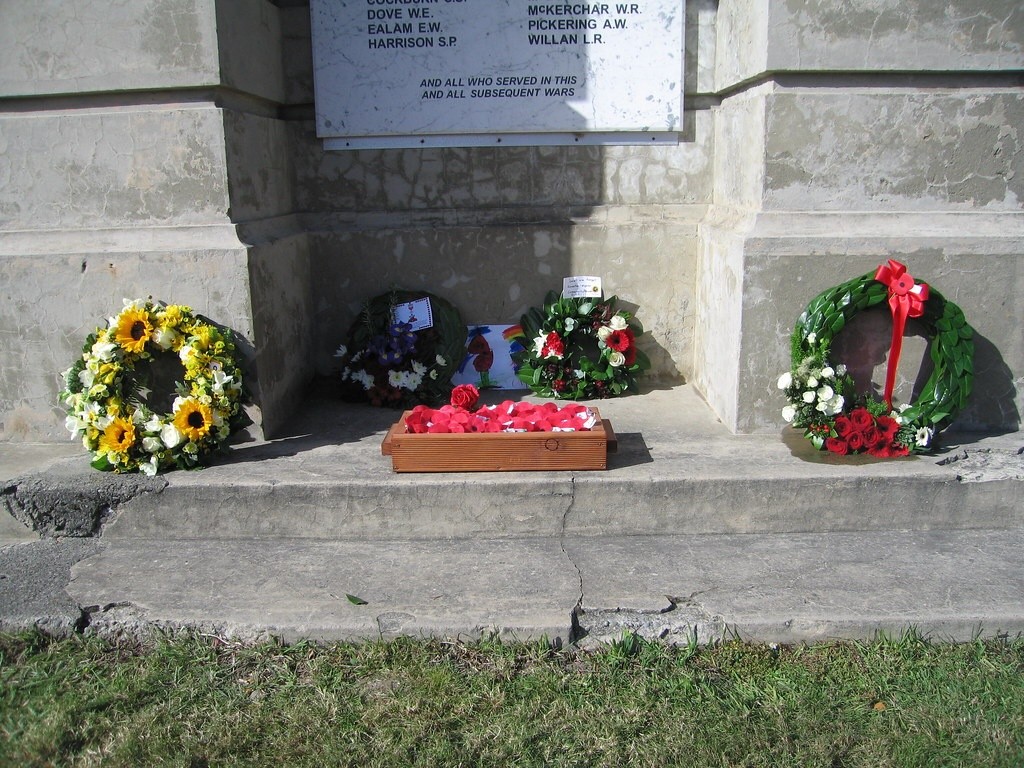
[332,288,467,410]
[404,400,596,433]
[509,285,652,402]
[55,295,253,479]
[449,383,480,411]
[778,264,976,459]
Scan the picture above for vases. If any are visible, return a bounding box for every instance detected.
[381,410,617,472]
